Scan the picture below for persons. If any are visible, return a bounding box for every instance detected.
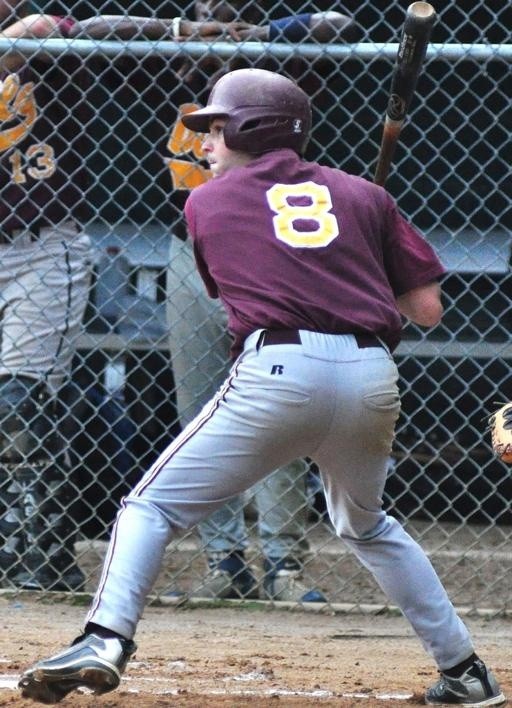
[0,0,98,590]
[20,66,508,708]
[64,0,362,603]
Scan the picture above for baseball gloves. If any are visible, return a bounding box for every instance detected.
[480,400,511,463]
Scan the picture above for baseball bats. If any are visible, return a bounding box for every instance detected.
[374,1,436,184]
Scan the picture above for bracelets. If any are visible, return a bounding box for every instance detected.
[266,12,312,44]
[172,16,182,39]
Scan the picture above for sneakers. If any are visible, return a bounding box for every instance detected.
[17,631,137,703]
[425,658,507,707]
[263,555,327,601]
[165,548,259,598]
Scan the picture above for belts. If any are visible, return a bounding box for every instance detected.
[0,226,39,244]
[258,328,383,348]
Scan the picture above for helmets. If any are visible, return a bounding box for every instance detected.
[181,67,313,158]
